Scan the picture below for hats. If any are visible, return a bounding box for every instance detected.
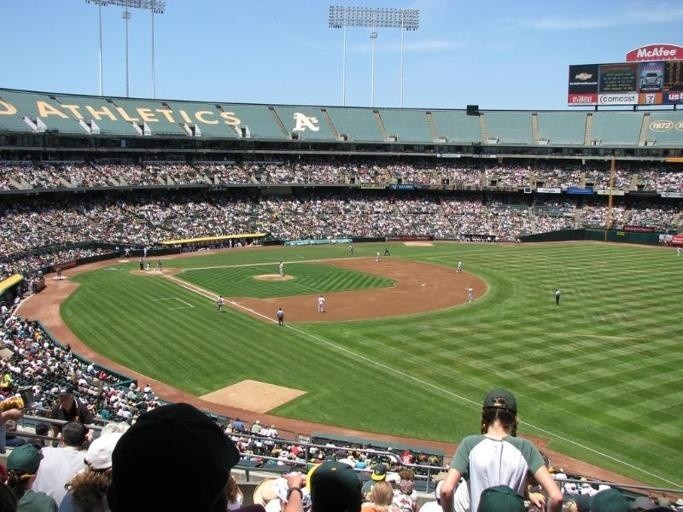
[483,388,517,411]
[477,485,524,511]
[371,465,386,480]
[590,488,630,511]
[112,404,240,511]
[85,433,126,470]
[252,475,280,506]
[305,461,363,503]
[7,444,44,475]
[59,389,72,396]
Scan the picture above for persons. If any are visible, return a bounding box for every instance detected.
[1,152,682,511]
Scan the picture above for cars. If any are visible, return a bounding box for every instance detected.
[640,73,662,87]
